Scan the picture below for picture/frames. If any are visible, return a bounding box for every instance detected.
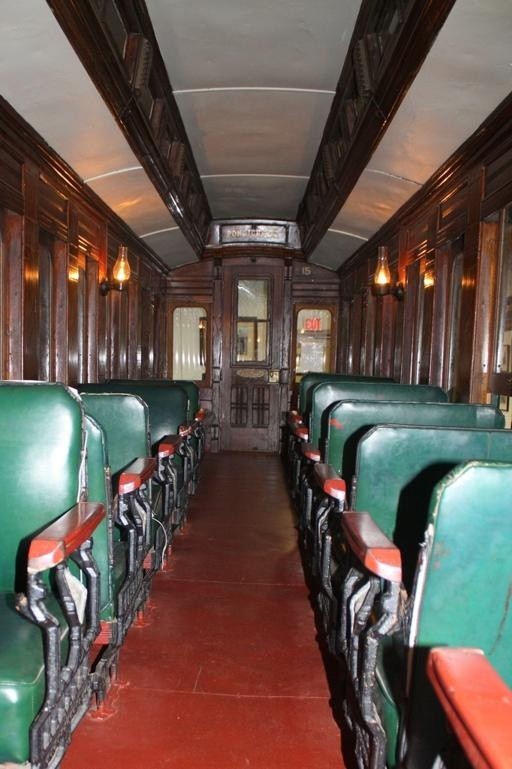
[371,246,405,302]
[236,316,257,362]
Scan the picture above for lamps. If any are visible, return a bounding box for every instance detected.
[99,244,132,297]
